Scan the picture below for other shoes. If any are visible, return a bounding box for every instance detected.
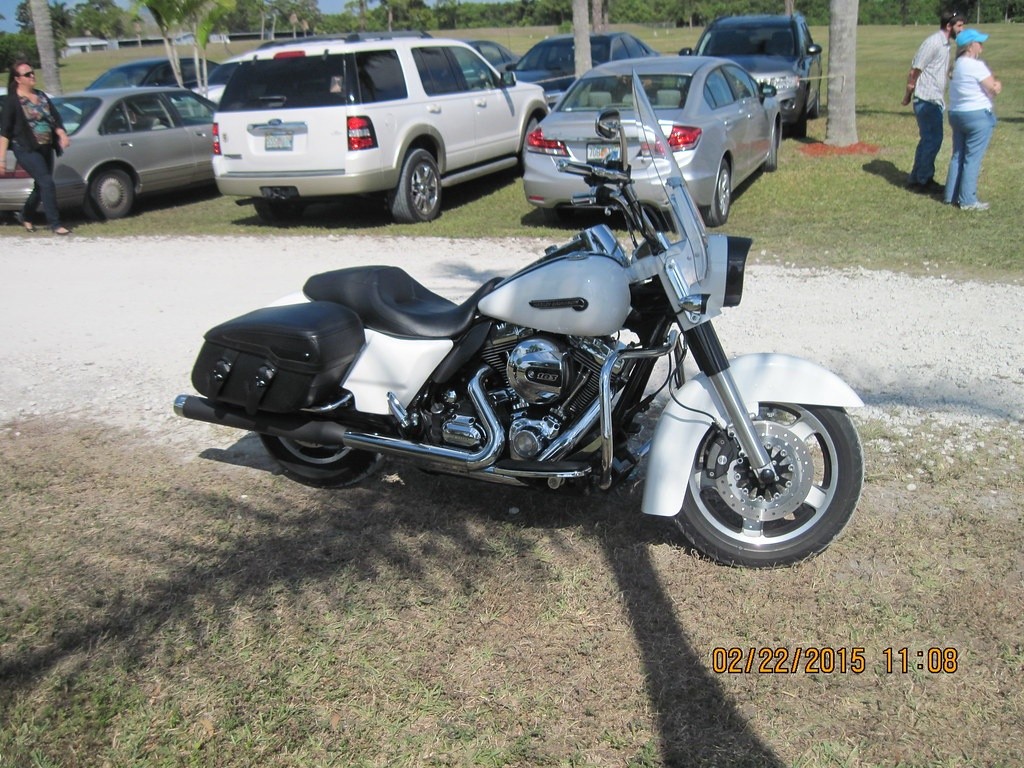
[54,227,70,235]
[20,218,36,232]
[910,180,945,193]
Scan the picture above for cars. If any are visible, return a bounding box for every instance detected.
[0,84,52,131]
[87,59,221,93]
[0,86,222,220]
[523,56,783,227]
[459,39,523,76]
[503,32,666,106]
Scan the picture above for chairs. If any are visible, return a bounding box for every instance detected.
[657,89,681,107]
[588,91,611,107]
[136,117,165,130]
[772,32,793,56]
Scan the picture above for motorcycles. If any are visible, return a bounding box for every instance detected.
[172,67,866,568]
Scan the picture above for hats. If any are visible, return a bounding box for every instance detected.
[956,30,988,47]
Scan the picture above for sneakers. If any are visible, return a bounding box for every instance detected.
[960,203,989,211]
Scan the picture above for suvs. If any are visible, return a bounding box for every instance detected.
[679,14,824,139]
[209,38,553,224]
[187,28,435,104]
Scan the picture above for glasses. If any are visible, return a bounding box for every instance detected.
[949,11,960,21]
[18,71,34,77]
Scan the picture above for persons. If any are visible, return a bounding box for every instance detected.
[902,9,964,193]
[1,59,70,236]
[944,28,1002,213]
